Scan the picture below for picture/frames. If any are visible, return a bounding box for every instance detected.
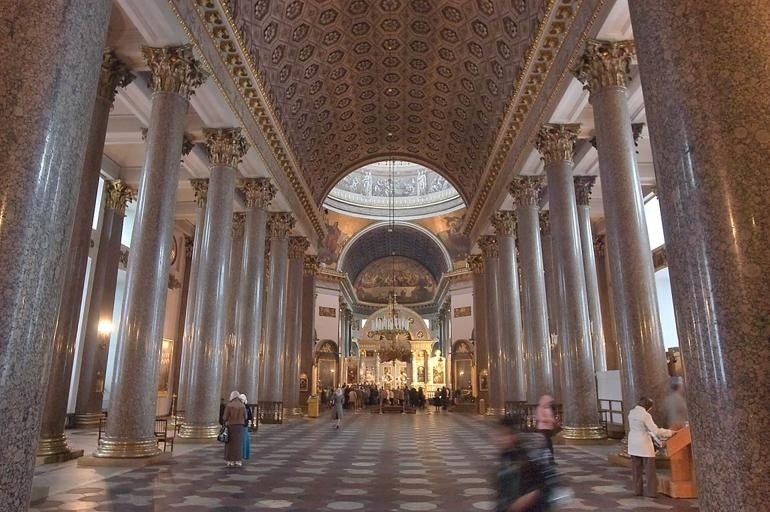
[157,337,175,397]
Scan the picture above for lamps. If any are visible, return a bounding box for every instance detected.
[373,253,412,366]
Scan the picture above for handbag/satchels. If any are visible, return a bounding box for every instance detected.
[328,399,335,409]
[217,422,229,444]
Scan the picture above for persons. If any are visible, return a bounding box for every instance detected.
[665,377,688,428]
[240,393,253,460]
[495,413,553,512]
[322,381,450,429]
[223,390,247,468]
[626,396,677,498]
[536,394,558,466]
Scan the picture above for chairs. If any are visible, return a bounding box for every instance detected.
[98,418,107,445]
[154,419,174,453]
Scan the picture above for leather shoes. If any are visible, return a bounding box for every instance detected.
[223,460,249,469]
